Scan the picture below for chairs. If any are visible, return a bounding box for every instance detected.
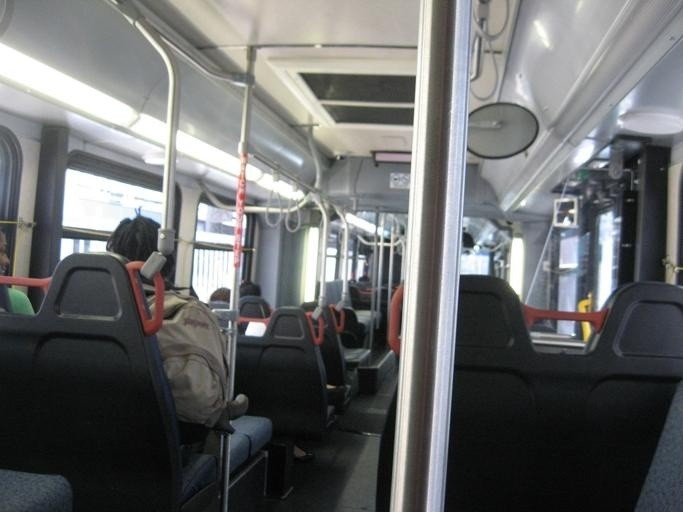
[0,252,683,512]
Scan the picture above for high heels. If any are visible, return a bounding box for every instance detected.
[216,393,249,434]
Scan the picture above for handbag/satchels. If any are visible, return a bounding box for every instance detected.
[156,299,229,429]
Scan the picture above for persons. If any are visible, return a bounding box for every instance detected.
[208,285,231,303]
[108,207,250,456]
[0,220,35,317]
[238,278,261,297]
